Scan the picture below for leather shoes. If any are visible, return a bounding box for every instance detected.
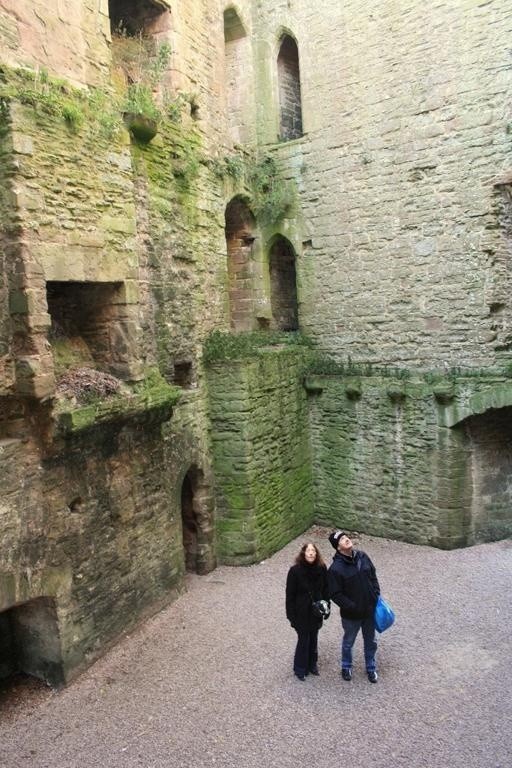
[297,670,320,681]
[341,668,352,681]
[368,670,378,683]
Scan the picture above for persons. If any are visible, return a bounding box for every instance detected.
[284,541,331,681]
[326,530,382,683]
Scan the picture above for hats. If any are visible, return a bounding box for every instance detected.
[329,532,345,549]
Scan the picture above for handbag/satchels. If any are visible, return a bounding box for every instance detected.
[312,600,331,618]
